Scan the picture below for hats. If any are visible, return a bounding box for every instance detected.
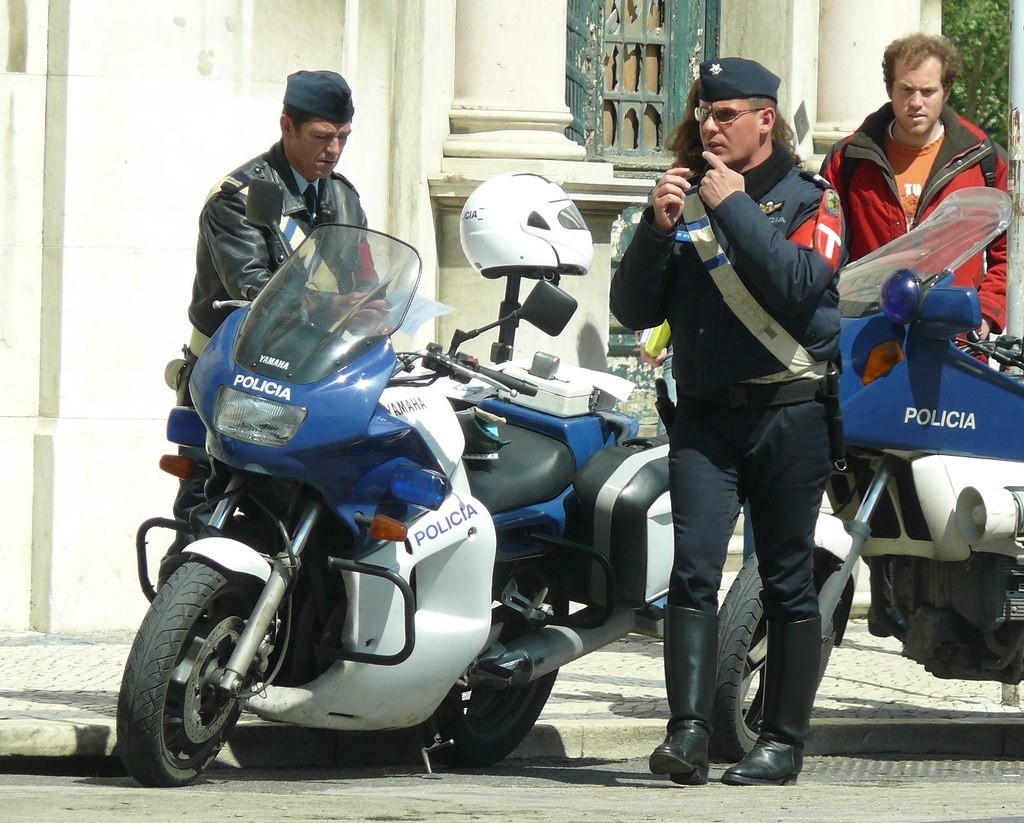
[698,56,781,103]
[284,70,354,124]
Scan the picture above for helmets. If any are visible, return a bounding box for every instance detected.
[458,171,595,280]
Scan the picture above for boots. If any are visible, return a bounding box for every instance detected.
[720,613,821,785]
[649,604,718,784]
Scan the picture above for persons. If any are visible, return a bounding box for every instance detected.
[608,55,847,789]
[173,70,390,540]
[818,34,1009,369]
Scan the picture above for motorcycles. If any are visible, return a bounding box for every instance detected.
[701,185,1022,761]
[112,225,640,793]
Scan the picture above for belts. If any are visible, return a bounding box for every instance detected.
[676,371,845,411]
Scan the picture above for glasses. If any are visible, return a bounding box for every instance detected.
[694,105,766,126]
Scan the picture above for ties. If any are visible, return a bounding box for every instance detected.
[303,184,318,227]
